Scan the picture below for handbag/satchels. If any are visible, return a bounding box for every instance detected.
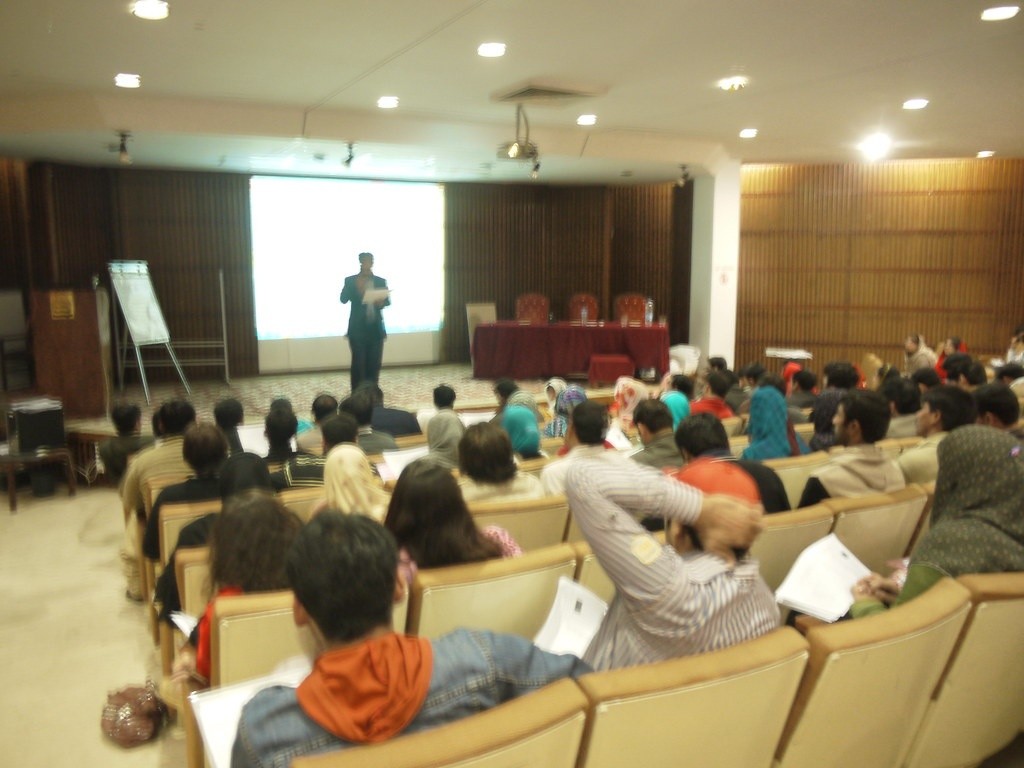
[101,682,170,749]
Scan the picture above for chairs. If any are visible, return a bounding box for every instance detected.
[613,292,652,323]
[513,293,550,319]
[568,294,600,321]
[108,360,1024,768]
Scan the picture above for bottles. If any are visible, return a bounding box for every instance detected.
[582,309,586,325]
[644,296,653,326]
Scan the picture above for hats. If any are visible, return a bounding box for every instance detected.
[738,366,763,377]
[662,452,762,510]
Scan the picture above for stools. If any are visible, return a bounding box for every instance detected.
[590,354,634,388]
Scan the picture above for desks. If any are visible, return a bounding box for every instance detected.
[473,321,670,383]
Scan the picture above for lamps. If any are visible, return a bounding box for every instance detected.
[343,143,357,167]
[501,101,536,161]
[677,164,689,187]
[529,164,540,181]
[117,135,135,166]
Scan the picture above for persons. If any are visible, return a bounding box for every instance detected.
[341,252,391,394]
[104,324,1024,768]
